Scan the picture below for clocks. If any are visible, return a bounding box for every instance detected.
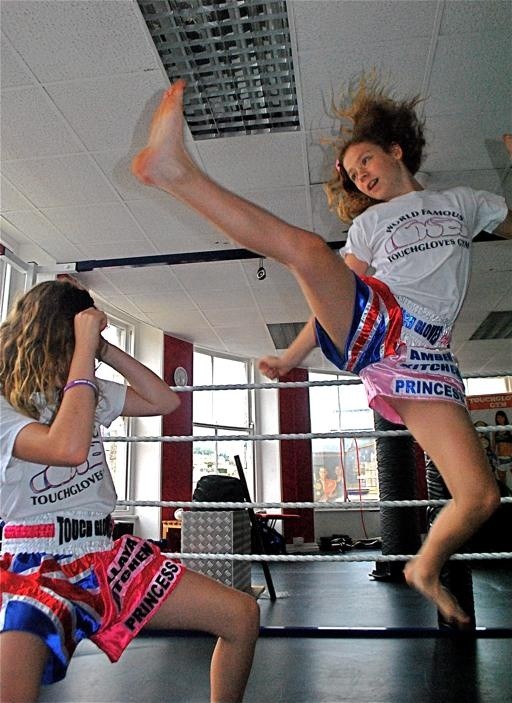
[174,367,188,386]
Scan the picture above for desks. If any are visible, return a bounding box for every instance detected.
[256,513,304,535]
[162,520,183,540]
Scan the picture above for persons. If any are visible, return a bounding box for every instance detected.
[491,411,511,484]
[1,281,262,703]
[316,463,344,502]
[126,62,511,623]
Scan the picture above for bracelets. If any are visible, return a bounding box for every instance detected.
[63,379,99,397]
